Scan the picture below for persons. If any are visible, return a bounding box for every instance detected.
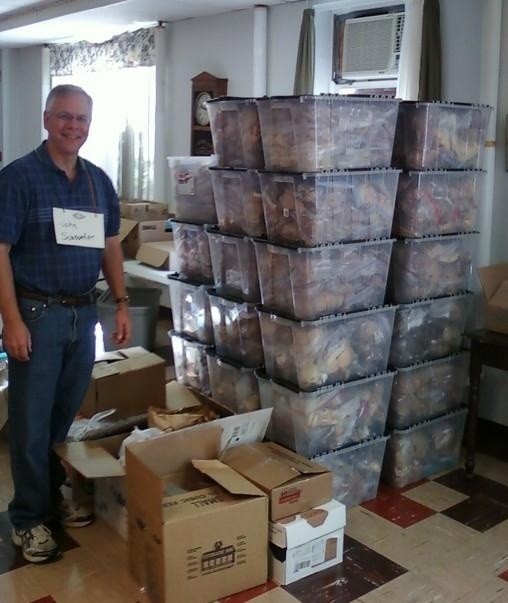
[1,84,131,564]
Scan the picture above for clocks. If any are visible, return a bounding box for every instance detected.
[193,92,212,123]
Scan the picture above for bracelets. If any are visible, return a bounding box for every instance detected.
[115,295,129,304]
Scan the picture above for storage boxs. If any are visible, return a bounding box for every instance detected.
[56,346,346,600]
[170,89,495,500]
[94,279,166,353]
[467,258,508,330]
[103,192,173,272]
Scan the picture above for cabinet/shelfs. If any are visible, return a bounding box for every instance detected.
[191,70,228,155]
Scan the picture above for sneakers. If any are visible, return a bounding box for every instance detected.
[11,523,59,563]
[60,498,95,528]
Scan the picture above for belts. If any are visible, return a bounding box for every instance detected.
[20,289,100,307]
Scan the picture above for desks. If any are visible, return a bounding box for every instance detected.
[459,329,507,481]
